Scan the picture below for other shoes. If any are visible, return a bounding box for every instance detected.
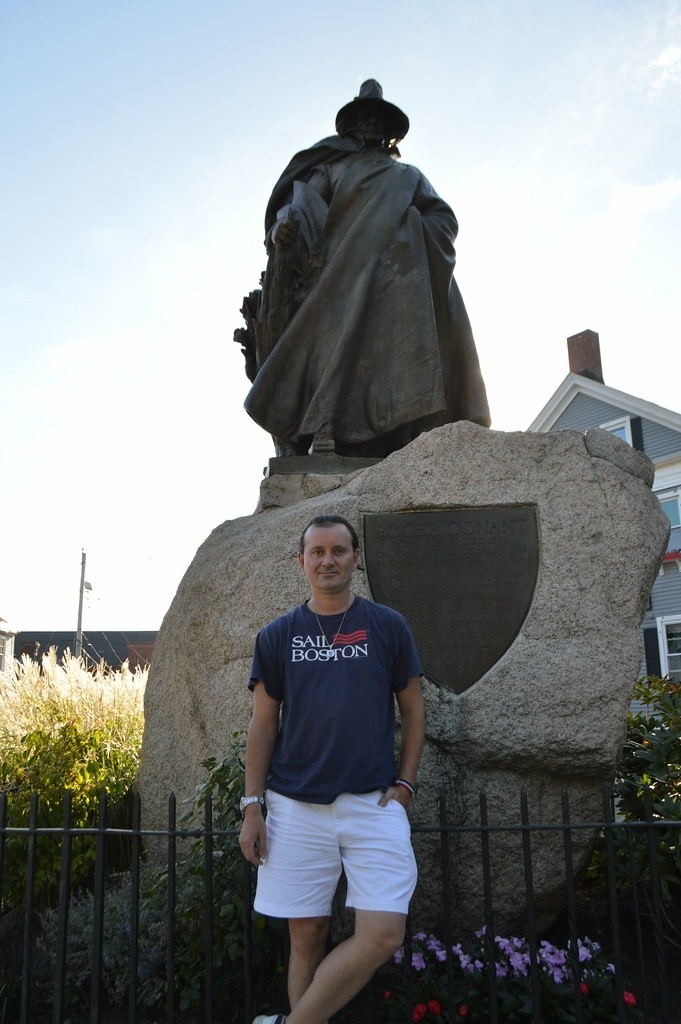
[252,1014,286,1024]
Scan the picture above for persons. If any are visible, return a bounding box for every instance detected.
[233,79,492,453]
[239,515,426,1024]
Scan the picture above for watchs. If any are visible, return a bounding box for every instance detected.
[240,795,265,812]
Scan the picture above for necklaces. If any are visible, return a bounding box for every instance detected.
[311,598,352,657]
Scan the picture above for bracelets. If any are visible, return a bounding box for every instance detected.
[395,778,418,797]
